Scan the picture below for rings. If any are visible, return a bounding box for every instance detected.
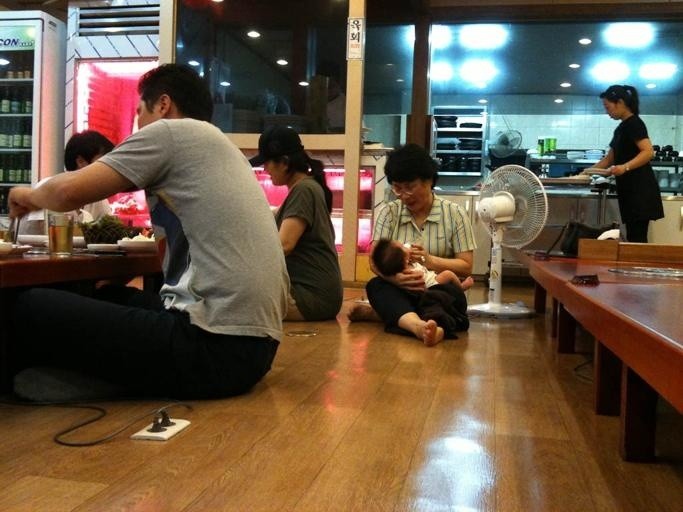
[419,255,425,264]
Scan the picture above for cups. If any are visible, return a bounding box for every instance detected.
[47,209,74,255]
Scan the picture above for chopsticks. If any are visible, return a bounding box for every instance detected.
[2,215,20,244]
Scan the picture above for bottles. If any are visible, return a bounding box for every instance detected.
[1,153,31,184]
[0,52,33,77]
[0,187,9,213]
[0,118,31,149]
[0,85,31,113]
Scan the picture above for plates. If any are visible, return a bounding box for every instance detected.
[566,149,602,159]
[118,238,155,251]
[434,114,457,127]
[17,235,48,246]
[87,243,117,252]
[459,122,482,126]
[10,245,32,256]
[584,167,611,177]
[437,137,480,149]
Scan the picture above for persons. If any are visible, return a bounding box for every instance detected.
[346,146,476,348]
[317,62,345,131]
[13,131,116,236]
[246,129,343,322]
[0,65,291,402]
[372,238,473,295]
[579,85,665,242]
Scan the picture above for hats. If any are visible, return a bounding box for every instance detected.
[248,125,303,167]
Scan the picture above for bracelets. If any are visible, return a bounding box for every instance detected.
[623,164,629,172]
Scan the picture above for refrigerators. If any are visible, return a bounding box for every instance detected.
[0,8,65,216]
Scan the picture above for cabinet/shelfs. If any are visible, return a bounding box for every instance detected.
[529,157,683,197]
[428,106,490,194]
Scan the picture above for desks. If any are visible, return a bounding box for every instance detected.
[520,240,681,511]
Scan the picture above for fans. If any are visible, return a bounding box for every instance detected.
[484,129,521,163]
[466,163,549,322]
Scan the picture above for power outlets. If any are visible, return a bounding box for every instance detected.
[131,416,192,441]
[355,297,369,303]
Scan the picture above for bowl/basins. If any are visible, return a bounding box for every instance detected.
[436,154,480,172]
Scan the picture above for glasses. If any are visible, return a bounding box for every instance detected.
[390,183,421,196]
